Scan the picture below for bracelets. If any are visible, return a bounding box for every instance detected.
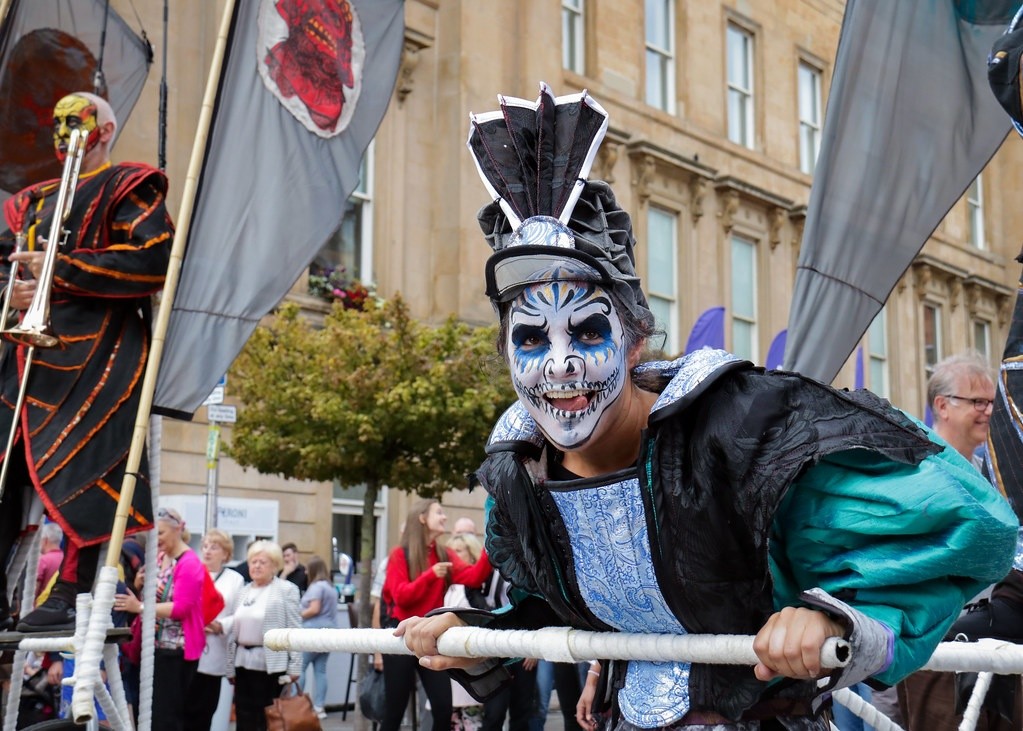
[587,670,600,676]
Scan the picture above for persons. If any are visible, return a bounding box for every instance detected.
[0,92,178,634]
[893,350,1011,731]
[113,506,338,731]
[370,500,603,731]
[394,83,1017,731]
[0,523,146,730]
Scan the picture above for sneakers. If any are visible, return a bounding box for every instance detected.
[0,593,14,630]
[15,580,83,631]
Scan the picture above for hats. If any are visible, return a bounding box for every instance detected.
[466,82,656,339]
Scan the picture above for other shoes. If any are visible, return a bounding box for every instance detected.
[314,705,328,719]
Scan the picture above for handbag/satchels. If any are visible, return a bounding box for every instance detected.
[120,616,142,667]
[265,679,323,731]
[359,668,389,724]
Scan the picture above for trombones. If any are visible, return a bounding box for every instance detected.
[0,128,90,501]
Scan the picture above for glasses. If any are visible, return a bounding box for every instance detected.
[944,395,996,413]
[159,507,180,526]
